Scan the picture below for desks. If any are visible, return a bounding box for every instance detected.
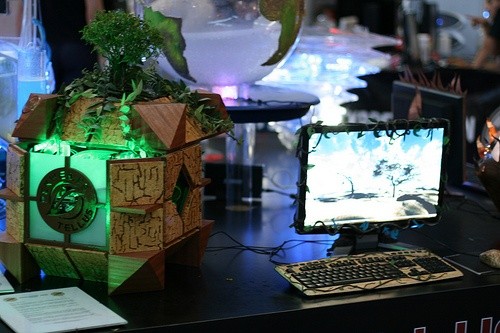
[0,27,500,333]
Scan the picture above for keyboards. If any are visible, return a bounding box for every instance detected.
[274,249,462,297]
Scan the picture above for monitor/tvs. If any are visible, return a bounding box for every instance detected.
[297,118,449,253]
[391,81,466,198]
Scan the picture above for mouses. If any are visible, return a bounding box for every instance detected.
[480,249,500,268]
